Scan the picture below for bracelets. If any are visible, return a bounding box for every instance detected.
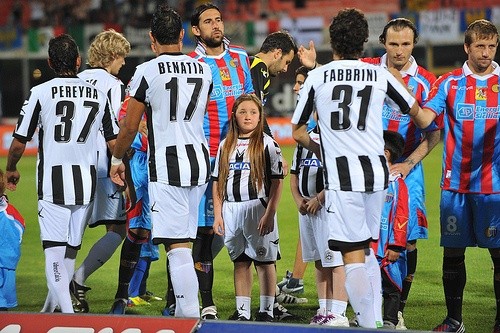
[310,63,316,70]
[111,156,123,166]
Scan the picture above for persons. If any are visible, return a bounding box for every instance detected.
[289,8,418,329]
[111,3,443,328]
[109,6,214,317]
[67,29,133,313]
[390,19,500,333]
[210,93,286,321]
[0,170,26,310]
[5,33,137,314]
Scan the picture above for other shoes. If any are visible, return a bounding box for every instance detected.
[108,300,128,317]
[256,310,275,322]
[162,304,175,318]
[228,311,253,322]
[380,320,397,330]
[396,310,407,331]
[201,306,219,320]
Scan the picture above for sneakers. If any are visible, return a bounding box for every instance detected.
[141,291,163,302]
[126,296,151,309]
[69,280,92,312]
[433,316,465,333]
[277,270,305,297]
[276,293,308,305]
[309,308,349,328]
[272,304,303,323]
